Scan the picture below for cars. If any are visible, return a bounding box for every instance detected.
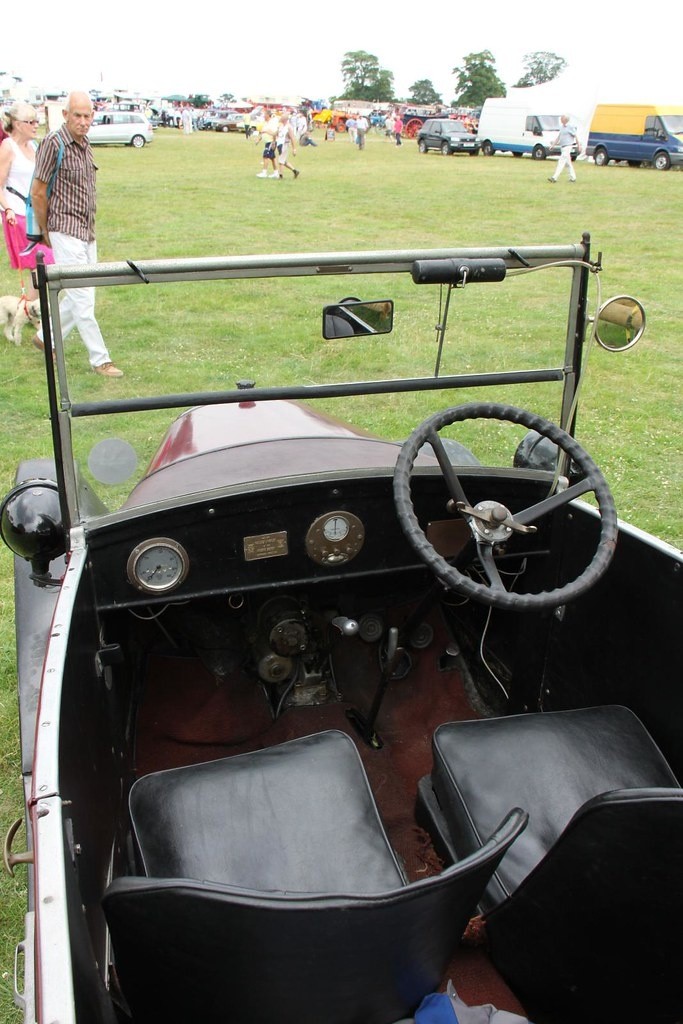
[166,109,256,132]
[106,101,160,130]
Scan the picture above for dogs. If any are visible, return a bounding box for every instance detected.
[0,296,50,345]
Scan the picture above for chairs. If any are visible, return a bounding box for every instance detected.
[103,115,110,124]
[99,728,530,1024]
[415,705,683,1024]
[325,314,354,338]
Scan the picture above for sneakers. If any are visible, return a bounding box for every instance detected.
[256,170,268,178]
[91,363,124,378]
[32,337,57,359]
[268,171,279,178]
[279,175,283,178]
[293,170,300,179]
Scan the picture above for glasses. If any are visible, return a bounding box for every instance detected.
[15,118,40,125]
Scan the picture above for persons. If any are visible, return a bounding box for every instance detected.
[0,91,124,377]
[243,108,252,138]
[162,106,198,135]
[547,115,581,183]
[300,113,403,149]
[255,110,300,178]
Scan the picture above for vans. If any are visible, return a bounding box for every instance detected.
[586,104,683,171]
[85,111,153,147]
[474,98,581,161]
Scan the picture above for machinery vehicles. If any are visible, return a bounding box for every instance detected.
[328,113,371,133]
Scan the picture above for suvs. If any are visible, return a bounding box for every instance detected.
[417,119,479,156]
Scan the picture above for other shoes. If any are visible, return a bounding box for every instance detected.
[567,180,576,183]
[547,178,556,182]
